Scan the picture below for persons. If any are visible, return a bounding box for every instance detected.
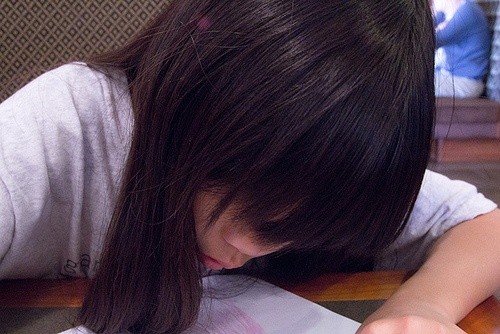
[1,1,500,334]
[432,1,490,97]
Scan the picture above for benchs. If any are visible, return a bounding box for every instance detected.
[430,0,500,162]
